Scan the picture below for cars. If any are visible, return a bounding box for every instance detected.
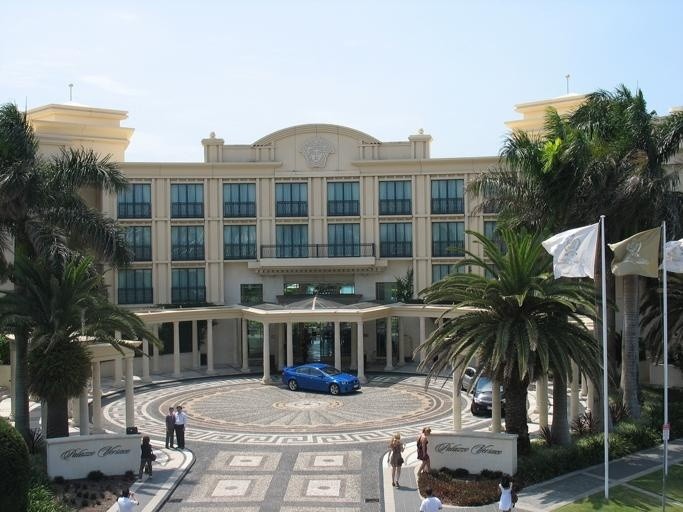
[281,362,361,395]
[460,366,506,415]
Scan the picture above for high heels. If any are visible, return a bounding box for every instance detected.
[392,481,400,489]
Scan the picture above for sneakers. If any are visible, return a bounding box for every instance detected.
[138,475,153,482]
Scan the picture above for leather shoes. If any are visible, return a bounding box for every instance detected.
[165,446,186,450]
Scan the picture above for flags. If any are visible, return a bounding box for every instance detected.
[656,236,682,274]
[540,221,599,281]
[606,226,658,280]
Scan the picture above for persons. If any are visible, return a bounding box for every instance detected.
[116,488,139,512]
[415,427,432,477]
[496,476,513,512]
[173,405,187,450]
[418,486,442,512]
[138,436,153,482]
[386,433,406,490]
[164,407,175,450]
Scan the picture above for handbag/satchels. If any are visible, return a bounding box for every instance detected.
[511,489,518,504]
[150,450,156,461]
